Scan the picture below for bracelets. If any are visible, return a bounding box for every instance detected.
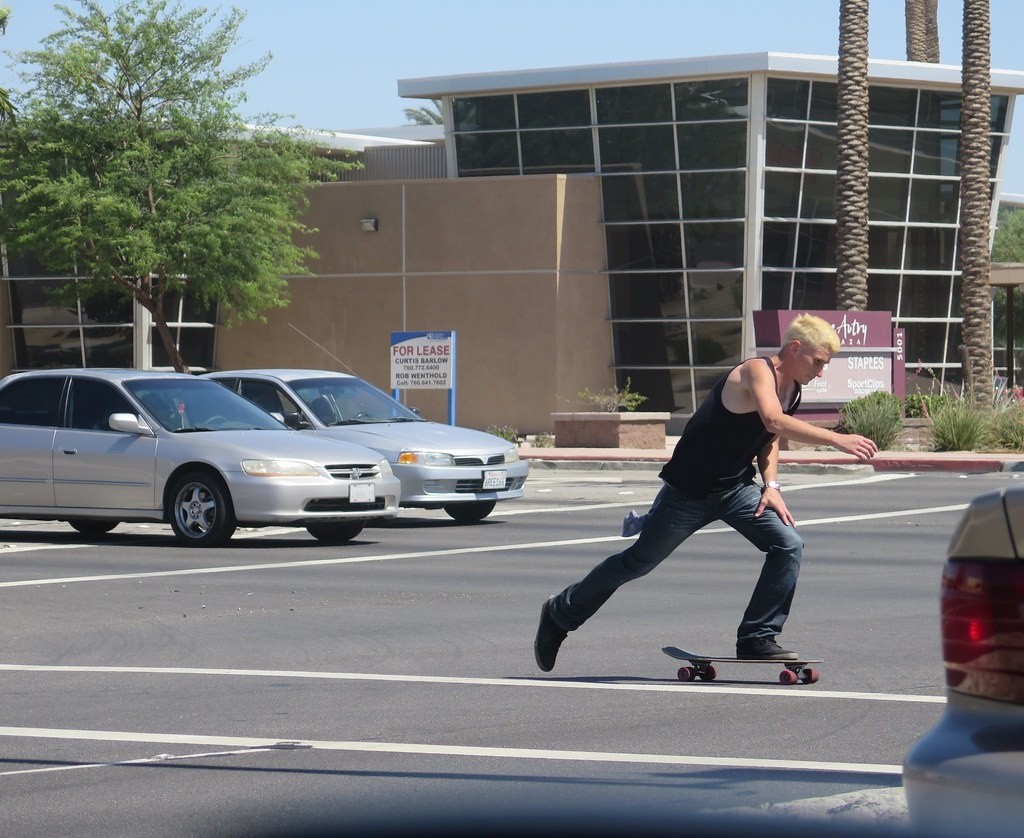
[764,482,780,491]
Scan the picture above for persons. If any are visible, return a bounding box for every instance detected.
[91,393,175,432]
[534,313,878,672]
[310,398,330,426]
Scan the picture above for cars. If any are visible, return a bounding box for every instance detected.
[197,368,530,523]
[0,368,401,547]
[904,487,1024,838]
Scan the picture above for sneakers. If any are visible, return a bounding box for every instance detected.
[735,632,799,660]
[533,595,568,672]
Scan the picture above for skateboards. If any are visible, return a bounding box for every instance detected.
[661,639,827,688]
